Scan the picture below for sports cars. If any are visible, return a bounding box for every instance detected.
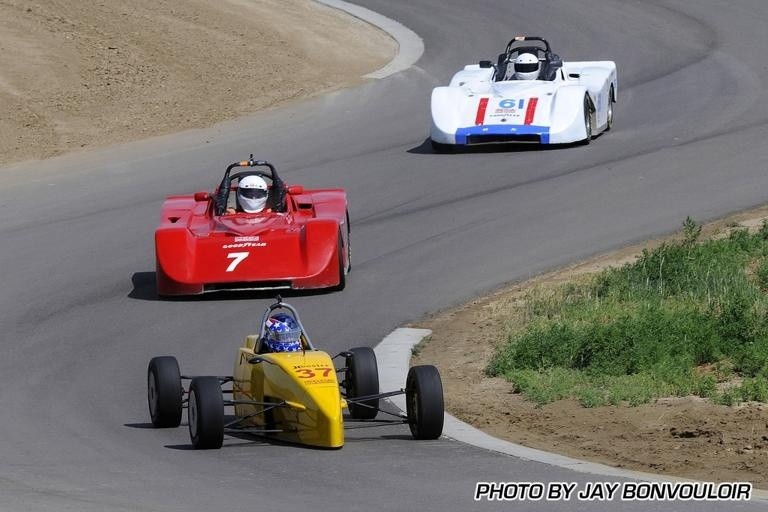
[430,34,617,149]
[155,157,352,300]
[146,295,446,448]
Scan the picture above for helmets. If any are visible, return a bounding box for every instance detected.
[514,53,540,80]
[262,314,302,352]
[237,175,268,213]
